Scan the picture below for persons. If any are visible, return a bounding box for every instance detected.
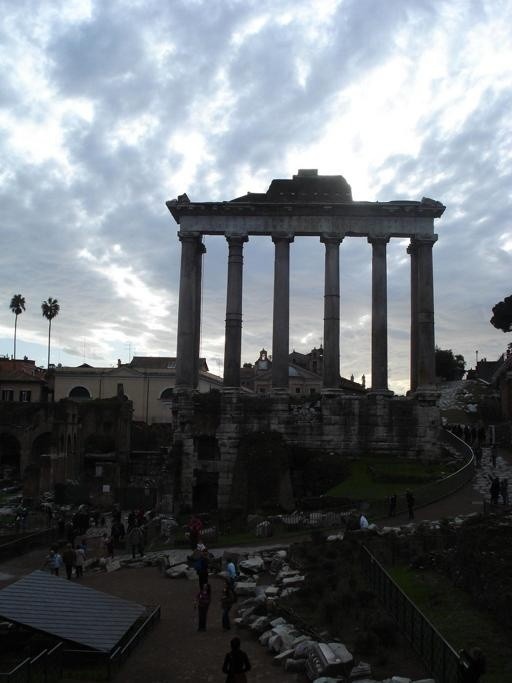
[197,550,209,589]
[226,557,236,588]
[45,503,145,579]
[448,423,497,468]
[457,646,489,682]
[192,519,199,549]
[222,635,252,682]
[488,473,500,504]
[499,478,507,504]
[189,514,203,539]
[195,582,212,631]
[406,487,415,519]
[217,582,235,629]
[385,494,397,516]
